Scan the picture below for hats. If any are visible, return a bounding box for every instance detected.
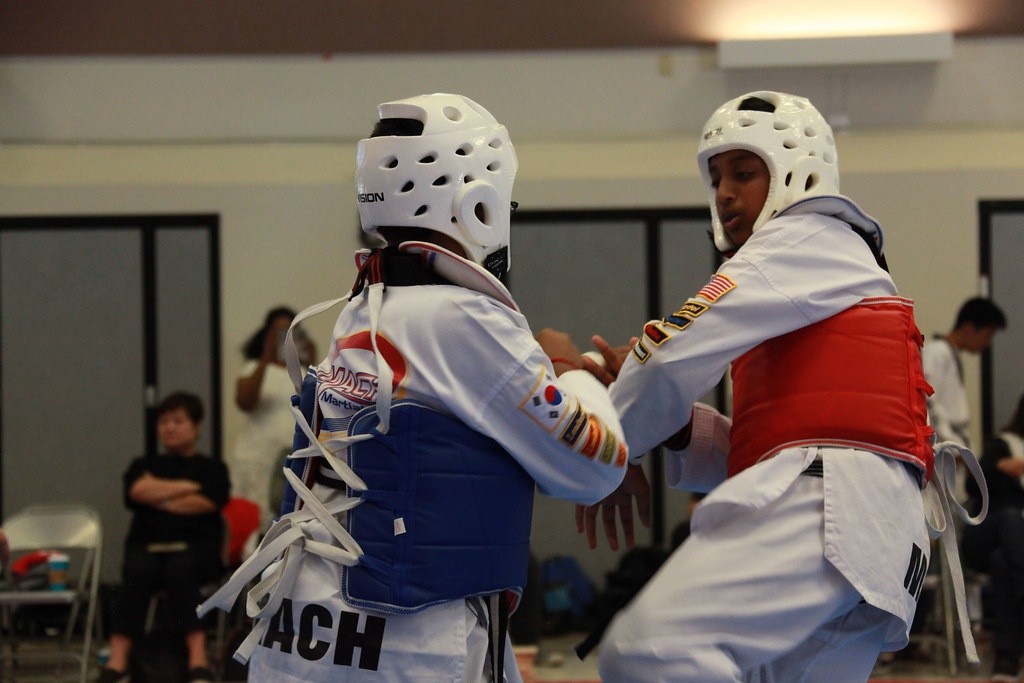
[695,90,841,253]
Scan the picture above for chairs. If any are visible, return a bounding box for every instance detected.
[145,498,261,661]
[0,502,105,683]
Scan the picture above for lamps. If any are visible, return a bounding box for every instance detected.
[715,30,956,71]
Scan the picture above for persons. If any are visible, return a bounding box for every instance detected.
[228,308,316,565]
[94,390,233,683]
[921,298,1007,637]
[579,91,935,683]
[962,392,1023,681]
[196,94,629,682]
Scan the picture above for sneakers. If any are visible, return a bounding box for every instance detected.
[184,666,217,683]
[989,643,1023,683]
[90,665,135,683]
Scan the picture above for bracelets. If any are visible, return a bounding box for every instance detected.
[551,357,577,367]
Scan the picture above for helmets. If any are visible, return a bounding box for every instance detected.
[353,90,520,282]
